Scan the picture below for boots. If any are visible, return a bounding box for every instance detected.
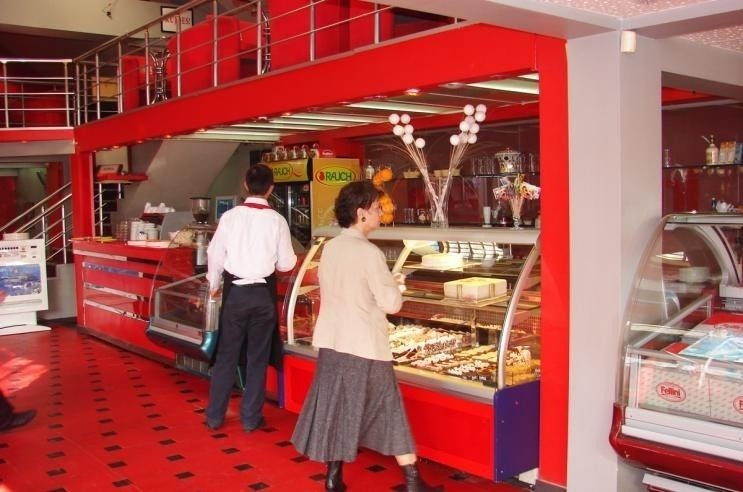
[325,460,346,491]
[399,462,423,492]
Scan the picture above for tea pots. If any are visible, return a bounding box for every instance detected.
[716,200,731,213]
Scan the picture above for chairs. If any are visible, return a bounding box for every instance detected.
[122,0,393,111]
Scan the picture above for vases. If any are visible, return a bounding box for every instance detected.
[509,199,525,230]
[423,177,454,229]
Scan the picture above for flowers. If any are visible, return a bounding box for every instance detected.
[493,173,541,201]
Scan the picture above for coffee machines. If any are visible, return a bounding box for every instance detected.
[189,196,212,274]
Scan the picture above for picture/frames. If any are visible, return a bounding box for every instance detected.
[215,196,236,224]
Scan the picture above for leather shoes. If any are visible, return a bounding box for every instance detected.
[1,409,36,431]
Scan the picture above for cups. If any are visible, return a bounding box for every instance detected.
[129,221,159,242]
[401,208,431,225]
[433,169,460,177]
[483,207,491,225]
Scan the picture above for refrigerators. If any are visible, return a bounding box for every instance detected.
[256,157,361,251]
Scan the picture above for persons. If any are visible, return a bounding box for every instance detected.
[291,178,441,492]
[0,390,36,431]
[199,162,298,431]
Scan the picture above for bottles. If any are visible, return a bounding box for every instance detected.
[711,197,716,212]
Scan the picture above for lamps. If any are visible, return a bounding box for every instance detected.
[621,31,636,54]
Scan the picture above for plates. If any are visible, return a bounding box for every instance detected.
[678,267,710,283]
